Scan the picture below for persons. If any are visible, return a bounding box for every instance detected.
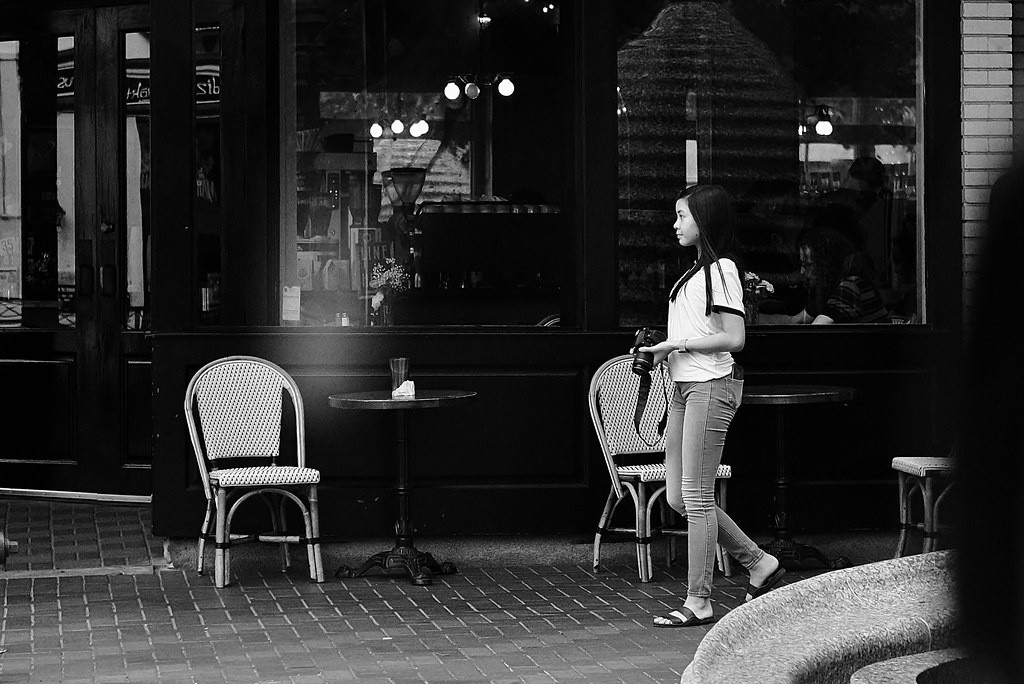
[627,179,779,627]
[754,138,895,325]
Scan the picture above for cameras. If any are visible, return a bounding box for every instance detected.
[632,327,667,376]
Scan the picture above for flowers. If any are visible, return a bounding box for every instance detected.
[372,257,410,292]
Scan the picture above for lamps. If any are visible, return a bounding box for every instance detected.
[813,105,833,136]
[442,71,515,100]
[369,95,429,139]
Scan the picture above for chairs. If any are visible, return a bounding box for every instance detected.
[184,356,325,588]
[588,354,732,585]
[892,444,960,559]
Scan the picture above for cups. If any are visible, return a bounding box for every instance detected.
[388,356,410,395]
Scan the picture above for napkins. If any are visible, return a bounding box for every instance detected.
[392,379,415,396]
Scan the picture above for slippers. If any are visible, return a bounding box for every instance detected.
[653,606,719,627]
[738,568,786,606]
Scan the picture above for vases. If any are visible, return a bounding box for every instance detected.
[379,293,394,326]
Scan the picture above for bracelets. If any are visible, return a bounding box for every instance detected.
[678,339,690,353]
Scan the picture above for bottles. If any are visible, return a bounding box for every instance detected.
[342,313,349,326]
[335,313,341,326]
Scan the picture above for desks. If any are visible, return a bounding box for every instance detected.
[328,390,479,586]
[741,386,853,571]
